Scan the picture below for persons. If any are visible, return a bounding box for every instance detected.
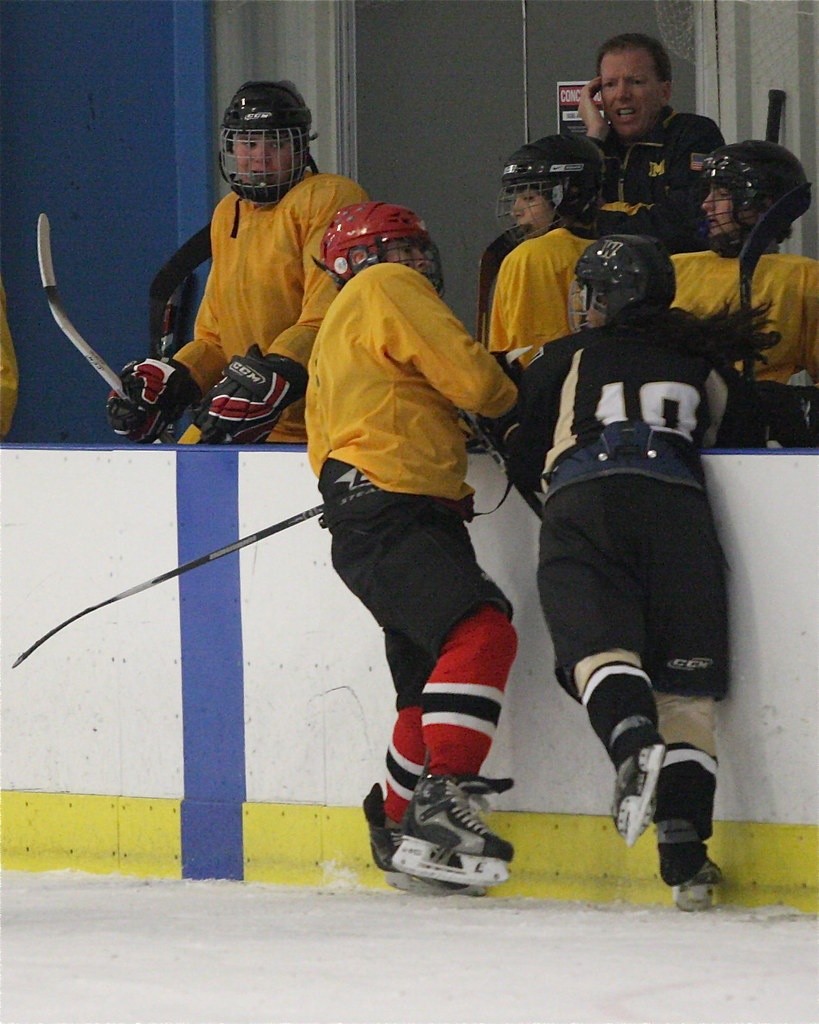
[578,33,727,258]
[516,235,818,912]
[304,202,518,896]
[669,140,819,449]
[106,80,372,444]
[477,134,611,372]
[0,271,19,444]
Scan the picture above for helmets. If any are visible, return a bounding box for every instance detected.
[217,82,314,203]
[565,234,677,337]
[702,137,813,257]
[496,136,606,242]
[324,201,446,293]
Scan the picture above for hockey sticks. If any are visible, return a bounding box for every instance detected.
[10,437,481,671]
[32,211,178,447]
[458,343,545,522]
[476,219,537,350]
[764,86,786,145]
[737,178,814,380]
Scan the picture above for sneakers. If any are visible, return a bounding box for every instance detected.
[611,729,665,845]
[654,821,724,911]
[361,784,491,896]
[390,753,515,883]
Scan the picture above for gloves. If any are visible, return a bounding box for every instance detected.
[190,341,309,448]
[475,382,532,449]
[106,356,196,443]
